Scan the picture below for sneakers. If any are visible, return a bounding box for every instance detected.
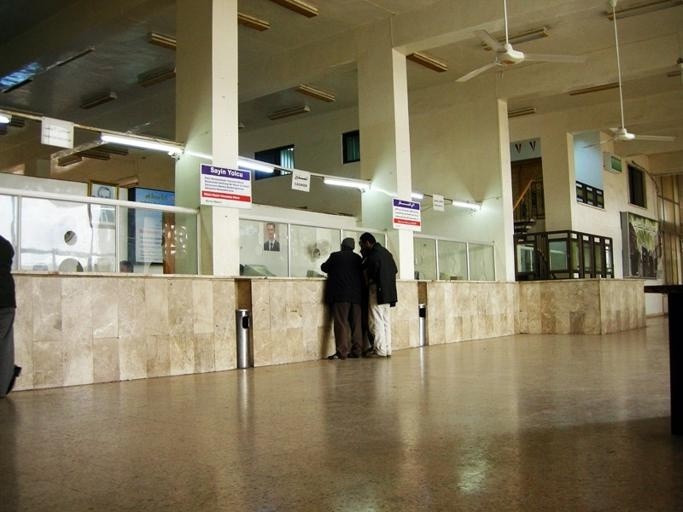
[328,349,391,359]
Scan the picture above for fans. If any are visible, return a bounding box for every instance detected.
[583,0,675,147]
[455,0,585,82]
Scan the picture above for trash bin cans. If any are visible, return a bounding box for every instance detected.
[236,309,250,369]
[419,304,426,347]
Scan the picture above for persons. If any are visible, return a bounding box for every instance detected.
[362,232,398,357]
[119,260,135,273]
[320,237,365,359]
[359,249,373,352]
[264,223,282,250]
[33,263,48,271]
[0,233,22,396]
